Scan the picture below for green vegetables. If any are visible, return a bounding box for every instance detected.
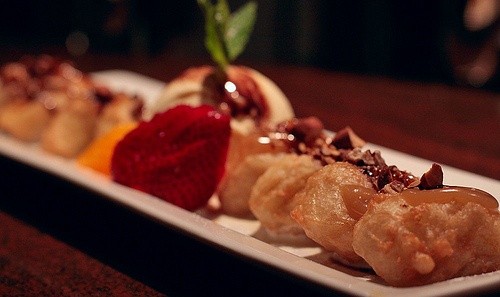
[200,0,258,71]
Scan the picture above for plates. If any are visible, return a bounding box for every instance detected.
[0,69,500,296]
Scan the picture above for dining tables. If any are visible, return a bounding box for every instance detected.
[0,54,500,297]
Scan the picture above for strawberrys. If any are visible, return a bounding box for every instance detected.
[111,102,231,214]
